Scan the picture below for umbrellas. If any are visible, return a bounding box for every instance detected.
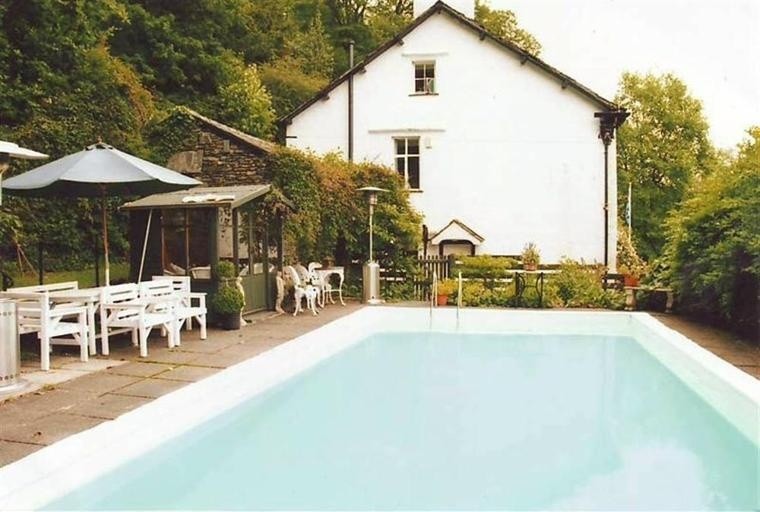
[1,136,204,286]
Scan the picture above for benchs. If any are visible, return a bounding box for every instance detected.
[621,286,679,313]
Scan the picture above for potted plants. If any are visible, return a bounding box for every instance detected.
[209,260,245,330]
[613,216,648,286]
[519,241,542,270]
[437,278,456,305]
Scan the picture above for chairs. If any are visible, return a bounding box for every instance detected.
[168,260,210,279]
[285,262,338,316]
[0,275,208,372]
[239,262,275,275]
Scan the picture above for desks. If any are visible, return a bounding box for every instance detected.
[314,266,346,308]
